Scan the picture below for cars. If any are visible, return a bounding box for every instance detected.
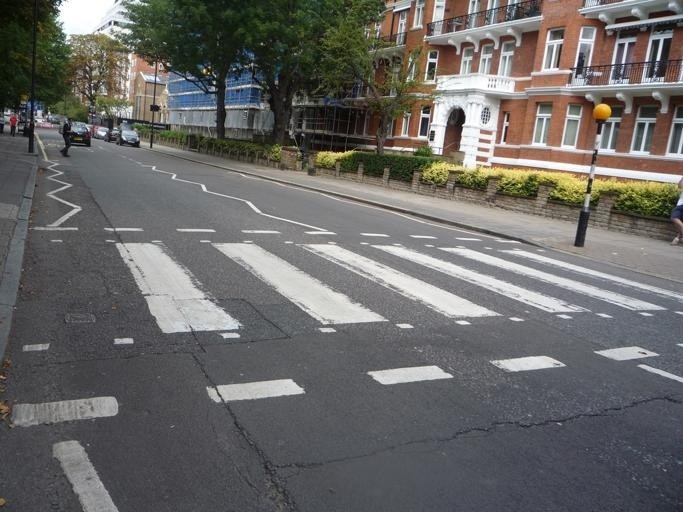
[47,115,140,147]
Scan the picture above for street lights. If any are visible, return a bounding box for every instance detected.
[574,104,611,246]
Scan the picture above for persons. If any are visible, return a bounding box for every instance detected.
[0,112,4,137]
[60,118,76,158]
[667,177,683,246]
[8,113,17,137]
